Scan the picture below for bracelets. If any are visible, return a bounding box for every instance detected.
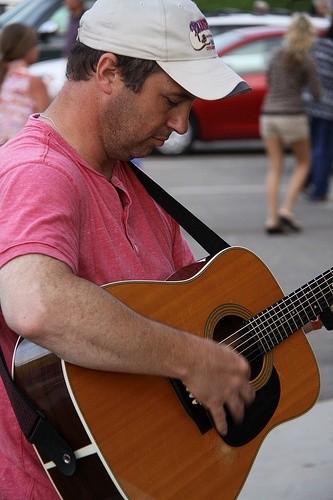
[311,316,322,330]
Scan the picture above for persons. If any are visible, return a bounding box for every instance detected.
[0,21,50,147]
[257,11,333,235]
[63,0,87,56]
[0,0,333,500]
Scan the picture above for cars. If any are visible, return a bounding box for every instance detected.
[154,25,329,158]
[0,0,98,66]
[27,14,333,108]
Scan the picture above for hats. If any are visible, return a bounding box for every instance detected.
[75,0,253,102]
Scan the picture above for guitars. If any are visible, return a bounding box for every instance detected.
[10,246,333,500]
